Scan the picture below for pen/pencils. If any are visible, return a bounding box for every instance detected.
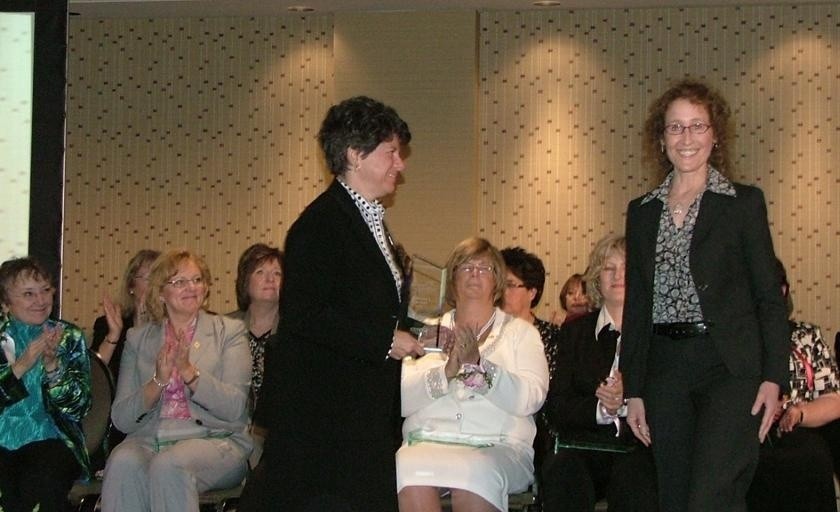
[435,313,441,348]
[412,331,422,360]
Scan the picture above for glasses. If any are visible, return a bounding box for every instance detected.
[449,261,494,280]
[502,281,529,289]
[162,275,206,289]
[659,120,713,138]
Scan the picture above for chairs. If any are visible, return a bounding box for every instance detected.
[52,349,117,511]
[93,381,269,512]
[439,482,546,512]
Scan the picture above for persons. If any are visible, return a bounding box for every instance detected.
[1,243,283,510]
[618,78,792,511]
[758,259,840,510]
[395,232,657,510]
[257,95,457,512]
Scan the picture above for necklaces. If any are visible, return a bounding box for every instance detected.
[669,195,696,214]
